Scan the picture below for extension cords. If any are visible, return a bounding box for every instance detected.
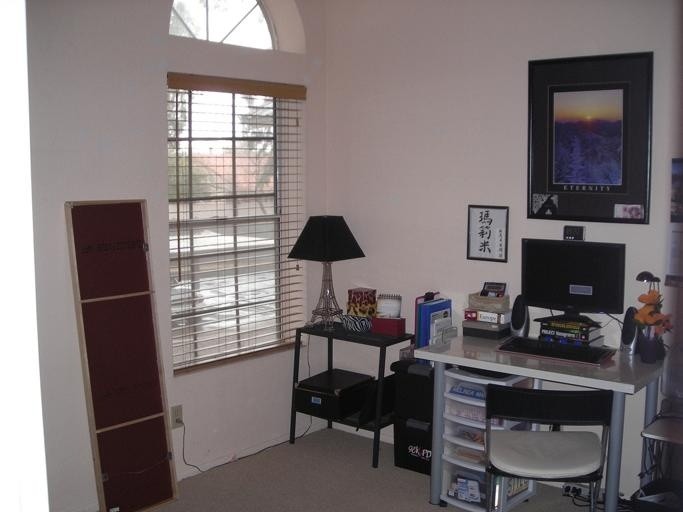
[562,483,590,498]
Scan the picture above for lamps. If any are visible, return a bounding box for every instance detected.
[635,271,660,290]
[285,214,366,333]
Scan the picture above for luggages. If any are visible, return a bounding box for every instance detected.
[293,368,374,420]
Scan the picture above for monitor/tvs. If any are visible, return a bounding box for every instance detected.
[521,237,626,328]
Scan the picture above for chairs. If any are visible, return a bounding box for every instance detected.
[480,382,614,512]
[637,343,682,512]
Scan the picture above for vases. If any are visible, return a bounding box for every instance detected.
[637,337,659,364]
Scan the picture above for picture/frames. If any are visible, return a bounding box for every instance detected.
[524,53,656,222]
[465,205,508,262]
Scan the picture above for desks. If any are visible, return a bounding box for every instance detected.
[414,332,664,512]
[289,320,414,472]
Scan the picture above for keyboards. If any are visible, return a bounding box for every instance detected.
[495,335,617,368]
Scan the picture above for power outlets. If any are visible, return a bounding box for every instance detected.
[170,404,185,429]
[559,482,620,507]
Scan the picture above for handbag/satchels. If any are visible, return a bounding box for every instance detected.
[358,374,397,425]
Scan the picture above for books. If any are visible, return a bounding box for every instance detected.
[415,291,451,365]
[538,321,604,348]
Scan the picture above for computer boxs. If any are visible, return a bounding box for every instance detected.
[389,371,432,476]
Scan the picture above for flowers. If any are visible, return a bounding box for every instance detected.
[629,291,676,338]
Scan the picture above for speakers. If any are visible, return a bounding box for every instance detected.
[619,306,638,356]
[509,295,529,338]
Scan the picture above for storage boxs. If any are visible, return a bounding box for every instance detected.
[439,368,535,512]
[391,358,432,473]
